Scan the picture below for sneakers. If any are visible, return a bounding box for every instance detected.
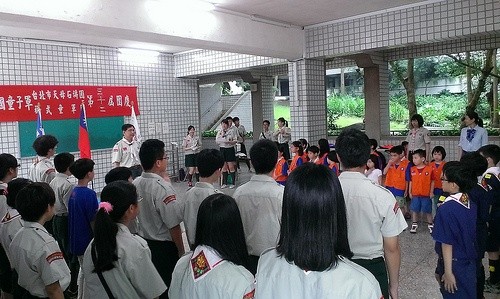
[428,223,435,232]
[410,223,418,232]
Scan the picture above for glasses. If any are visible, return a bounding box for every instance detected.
[138,195,143,202]
[155,156,169,163]
[440,176,449,181]
[16,164,20,168]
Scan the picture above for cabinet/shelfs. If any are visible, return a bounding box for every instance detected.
[165,142,181,183]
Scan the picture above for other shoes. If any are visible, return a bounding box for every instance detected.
[485,277,494,289]
[237,169,241,173]
[249,168,255,172]
[221,184,227,188]
[230,185,235,188]
[188,182,192,186]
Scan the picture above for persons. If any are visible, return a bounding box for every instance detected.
[433,161,477,299]
[131,139,185,299]
[254,162,384,299]
[168,193,256,299]
[77,181,168,299]
[175,148,225,251]
[258,111,500,299]
[182,126,201,187]
[9,182,71,299]
[215,116,255,189]
[338,126,408,299]
[231,140,284,275]
[0,135,134,299]
[111,124,142,181]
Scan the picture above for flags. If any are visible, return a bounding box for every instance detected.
[78,104,91,159]
[131,106,142,141]
[36,111,45,138]
